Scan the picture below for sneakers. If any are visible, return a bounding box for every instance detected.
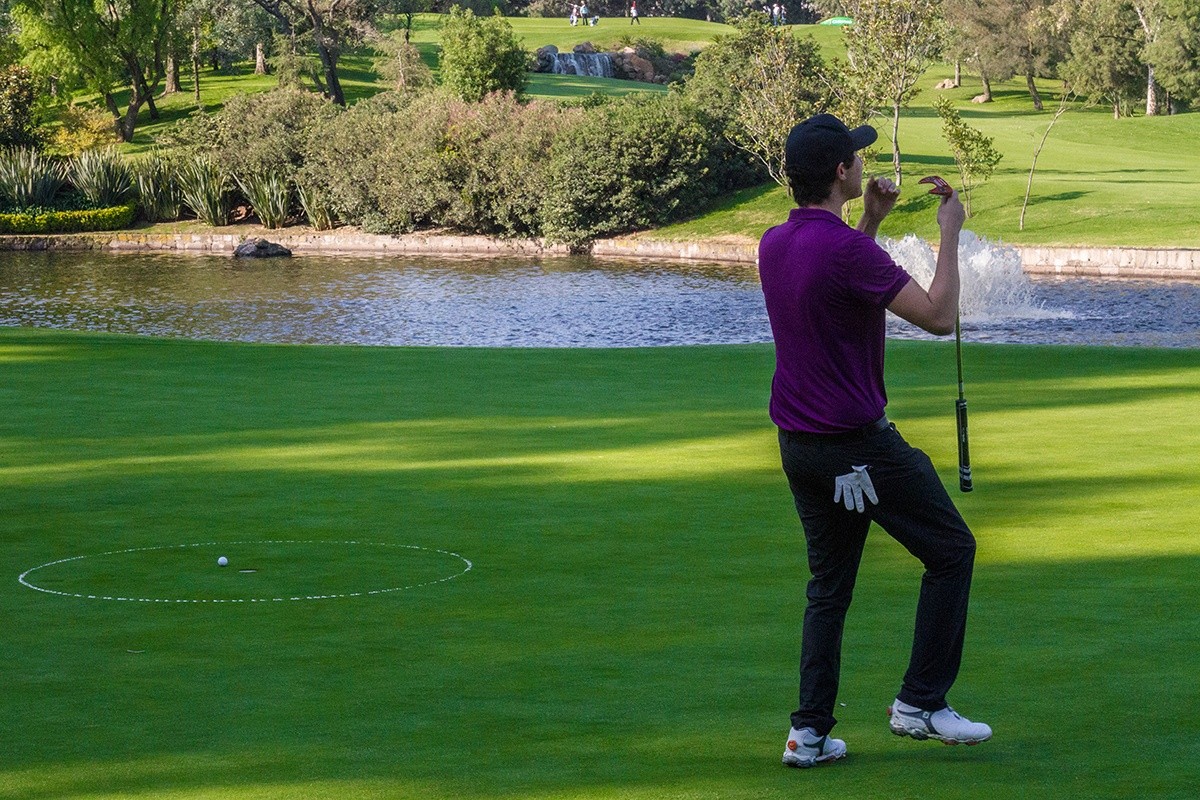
[888,698,993,745]
[782,726,847,768]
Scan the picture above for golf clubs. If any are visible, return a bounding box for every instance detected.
[916,174,975,493]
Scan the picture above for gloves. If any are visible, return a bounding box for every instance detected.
[833,466,879,513]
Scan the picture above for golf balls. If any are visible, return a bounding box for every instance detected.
[217,556,229,567]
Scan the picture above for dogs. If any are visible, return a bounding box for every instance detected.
[588,15,599,26]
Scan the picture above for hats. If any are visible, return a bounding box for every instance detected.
[785,114,878,172]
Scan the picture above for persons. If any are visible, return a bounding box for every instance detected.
[570,1,641,26]
[763,4,787,26]
[761,114,993,764]
